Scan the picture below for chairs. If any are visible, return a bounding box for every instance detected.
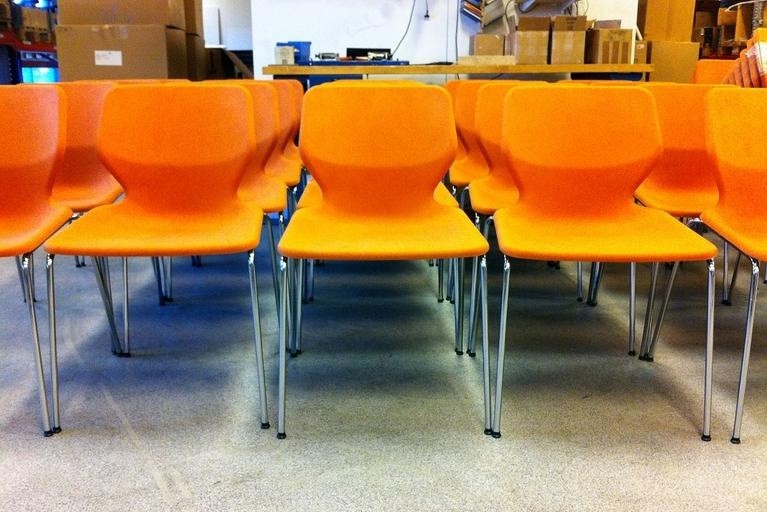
[75,80,193,308]
[721,27,767,88]
[20,80,125,355]
[166,82,288,303]
[691,58,739,84]
[193,81,307,213]
[558,80,635,84]
[637,84,742,361]
[295,82,460,355]
[445,80,550,103]
[51,84,280,435]
[192,79,308,268]
[276,85,493,440]
[465,83,637,356]
[492,85,713,442]
[449,82,548,203]
[0,84,74,437]
[645,87,766,449]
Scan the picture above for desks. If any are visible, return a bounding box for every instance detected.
[262,62,654,78]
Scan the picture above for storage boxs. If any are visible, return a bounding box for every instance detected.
[506,14,551,66]
[633,40,648,65]
[185,33,206,82]
[204,46,254,80]
[57,0,187,32]
[469,34,506,56]
[55,24,188,83]
[551,14,596,65]
[184,0,205,40]
[583,19,633,65]
